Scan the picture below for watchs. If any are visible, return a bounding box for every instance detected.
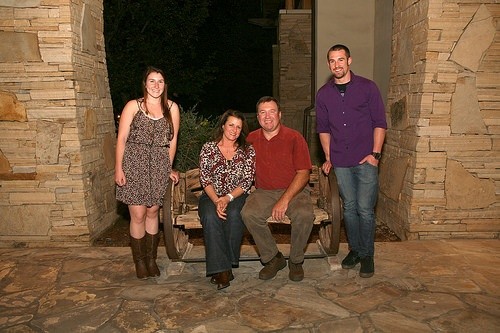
[370,152,382,160]
[227,194,234,202]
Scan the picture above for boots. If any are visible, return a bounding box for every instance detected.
[129,233,149,280]
[145,231,161,278]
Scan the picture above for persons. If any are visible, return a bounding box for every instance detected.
[316,44,387,278]
[197,110,256,290]
[115,66,180,280]
[240,97,314,282]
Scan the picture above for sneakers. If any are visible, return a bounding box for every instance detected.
[342,250,360,269]
[259,252,287,280]
[360,255,375,278]
[288,257,304,281]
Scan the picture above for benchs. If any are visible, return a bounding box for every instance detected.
[161,165,341,263]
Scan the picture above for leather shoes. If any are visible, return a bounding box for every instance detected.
[210,270,235,290]
